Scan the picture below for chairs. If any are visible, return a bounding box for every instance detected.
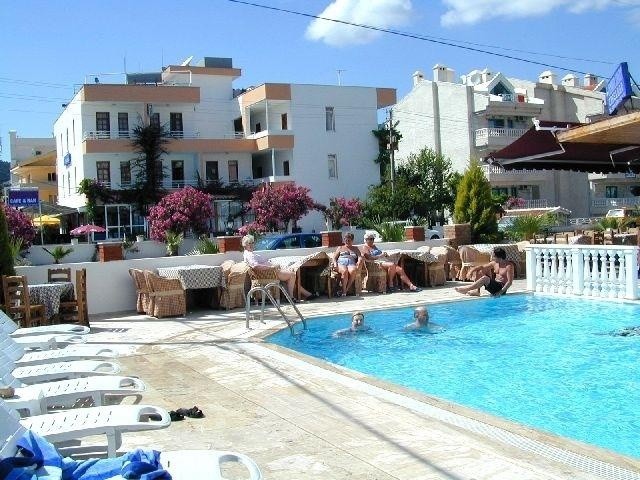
[445,226,640,282]
[0,266,94,328]
[219,258,249,310]
[0,308,148,416]
[128,266,189,321]
[247,243,449,306]
[0,406,262,480]
[0,398,172,465]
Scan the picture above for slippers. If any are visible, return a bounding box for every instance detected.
[287,297,301,303]
[306,293,319,300]
[151,411,184,421]
[390,286,394,292]
[176,407,203,418]
[410,287,422,291]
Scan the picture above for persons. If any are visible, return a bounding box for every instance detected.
[362,232,421,292]
[456,247,514,296]
[334,232,362,297]
[241,234,321,304]
[333,312,372,338]
[403,306,443,328]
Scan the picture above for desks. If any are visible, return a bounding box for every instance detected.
[156,263,222,311]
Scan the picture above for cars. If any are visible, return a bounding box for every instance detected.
[497,216,517,233]
[362,221,443,243]
[253,233,322,251]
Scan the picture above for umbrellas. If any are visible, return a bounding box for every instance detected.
[69,223,110,244]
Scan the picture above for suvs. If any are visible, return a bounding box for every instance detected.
[606,207,640,229]
